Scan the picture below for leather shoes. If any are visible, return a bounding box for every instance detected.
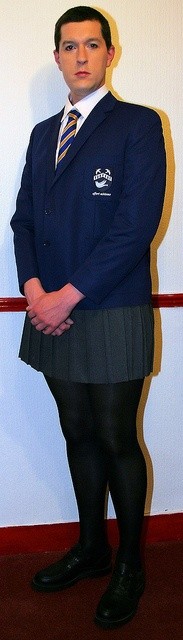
[93,549,146,628]
[29,549,106,595]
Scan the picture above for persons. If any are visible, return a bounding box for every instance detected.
[11,7,166,629]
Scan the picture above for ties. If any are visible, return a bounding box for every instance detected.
[57,109,85,167]
